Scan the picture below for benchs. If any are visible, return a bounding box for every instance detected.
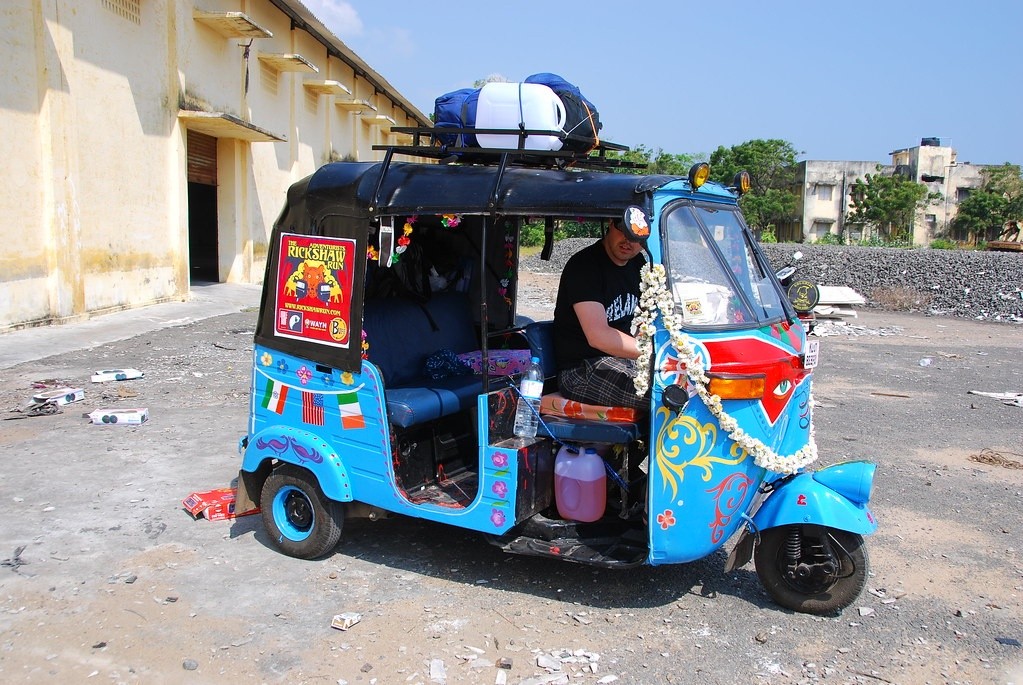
[363,290,530,487]
[521,320,646,519]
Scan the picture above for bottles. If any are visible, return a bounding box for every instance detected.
[920,358,933,367]
[513,356,544,439]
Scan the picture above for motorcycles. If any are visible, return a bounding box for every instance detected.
[238,123,879,615]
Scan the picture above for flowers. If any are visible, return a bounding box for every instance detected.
[362,214,462,358]
[500,221,513,305]
[631,263,818,476]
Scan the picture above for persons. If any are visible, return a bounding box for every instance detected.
[552,220,648,526]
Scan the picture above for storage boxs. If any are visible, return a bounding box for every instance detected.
[90,368,144,382]
[29,387,86,405]
[82,408,149,425]
[183,487,260,522]
[454,349,531,375]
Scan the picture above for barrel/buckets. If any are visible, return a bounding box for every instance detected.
[555,445,607,524]
[474,82,567,151]
